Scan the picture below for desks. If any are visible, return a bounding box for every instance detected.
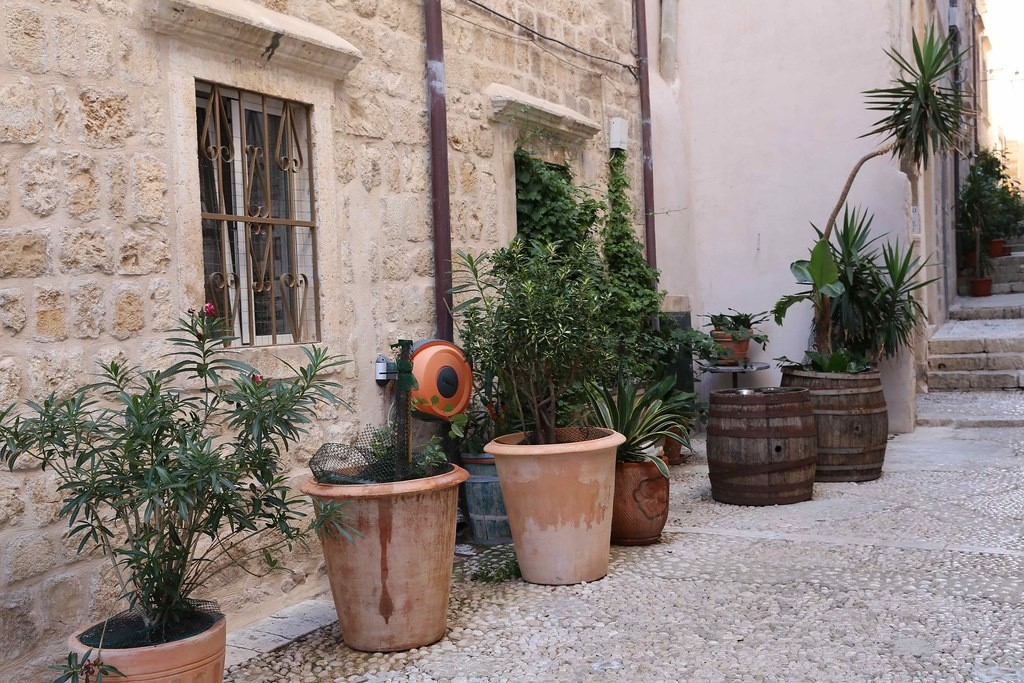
[700,362,770,387]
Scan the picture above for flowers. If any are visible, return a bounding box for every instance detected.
[0,303,366,683]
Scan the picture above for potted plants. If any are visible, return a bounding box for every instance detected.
[951,165,1019,297]
[770,237,889,482]
[696,307,770,365]
[566,377,696,544]
[440,236,669,585]
[648,374,697,466]
[301,360,470,655]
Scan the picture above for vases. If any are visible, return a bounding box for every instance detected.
[67,607,226,683]
[707,389,817,508]
